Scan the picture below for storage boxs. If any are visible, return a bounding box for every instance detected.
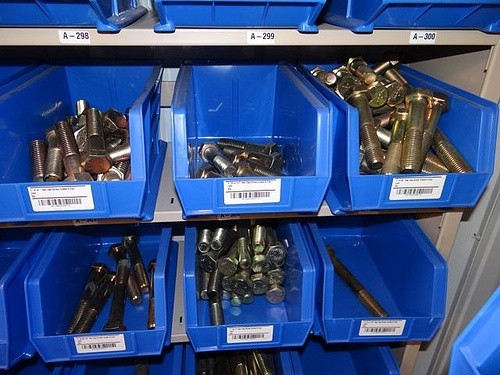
[0,0,500,375]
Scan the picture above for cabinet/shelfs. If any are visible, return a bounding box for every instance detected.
[1,27,500,374]
